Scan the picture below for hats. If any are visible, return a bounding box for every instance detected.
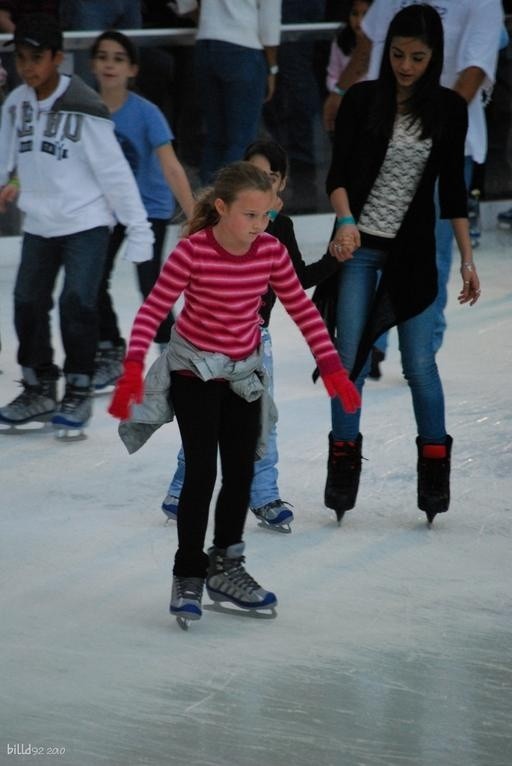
[3,14,63,53]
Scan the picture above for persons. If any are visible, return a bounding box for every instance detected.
[299,0,482,512]
[108,159,361,623]
[87,29,196,388]
[161,137,361,526]
[0,0,370,200]
[1,9,158,426]
[340,0,503,253]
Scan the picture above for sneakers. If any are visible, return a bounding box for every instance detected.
[162,494,180,517]
[252,499,293,527]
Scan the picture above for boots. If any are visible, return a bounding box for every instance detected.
[170,575,204,620]
[414,436,453,515]
[91,339,126,390]
[468,199,479,236]
[50,372,93,426]
[324,431,364,512]
[0,366,60,425]
[206,541,277,610]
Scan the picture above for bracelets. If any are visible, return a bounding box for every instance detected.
[335,214,357,228]
[459,260,477,274]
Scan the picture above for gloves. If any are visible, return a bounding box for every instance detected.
[321,369,362,414]
[108,362,146,422]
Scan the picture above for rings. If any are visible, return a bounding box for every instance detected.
[333,239,345,253]
